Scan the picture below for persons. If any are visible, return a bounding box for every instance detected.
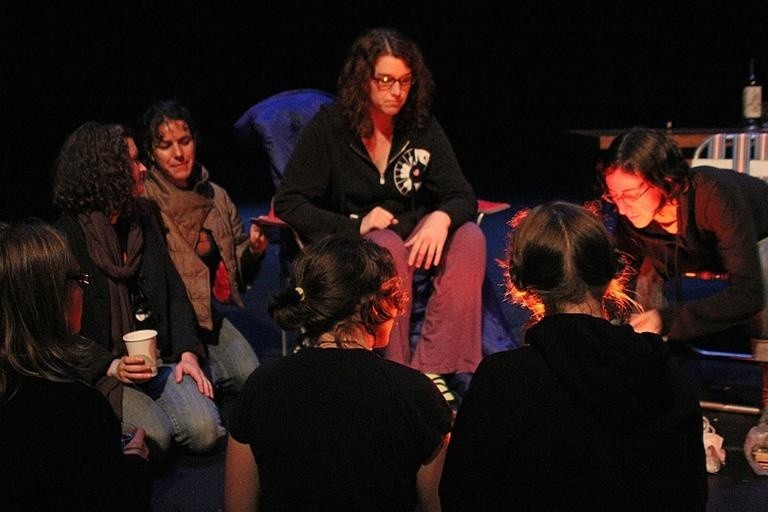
[225,232,453,511]
[135,100,271,394]
[435,200,710,511]
[272,24,489,413]
[50,121,228,455]
[597,126,768,348]
[0,219,157,511]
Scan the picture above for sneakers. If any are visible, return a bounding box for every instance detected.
[429,375,460,420]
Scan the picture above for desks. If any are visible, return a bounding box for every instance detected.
[562,127,756,171]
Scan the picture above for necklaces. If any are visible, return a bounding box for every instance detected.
[316,339,368,349]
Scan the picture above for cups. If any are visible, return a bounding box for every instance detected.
[124,326,159,378]
[754,132,768,162]
[707,132,726,160]
[732,133,751,176]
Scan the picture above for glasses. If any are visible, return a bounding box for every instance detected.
[600,185,652,207]
[69,270,91,289]
[372,71,413,89]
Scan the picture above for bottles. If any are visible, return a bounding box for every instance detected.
[741,60,764,131]
[743,409,768,481]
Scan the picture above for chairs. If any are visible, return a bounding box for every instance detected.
[234,86,510,359]
[683,165,768,417]
